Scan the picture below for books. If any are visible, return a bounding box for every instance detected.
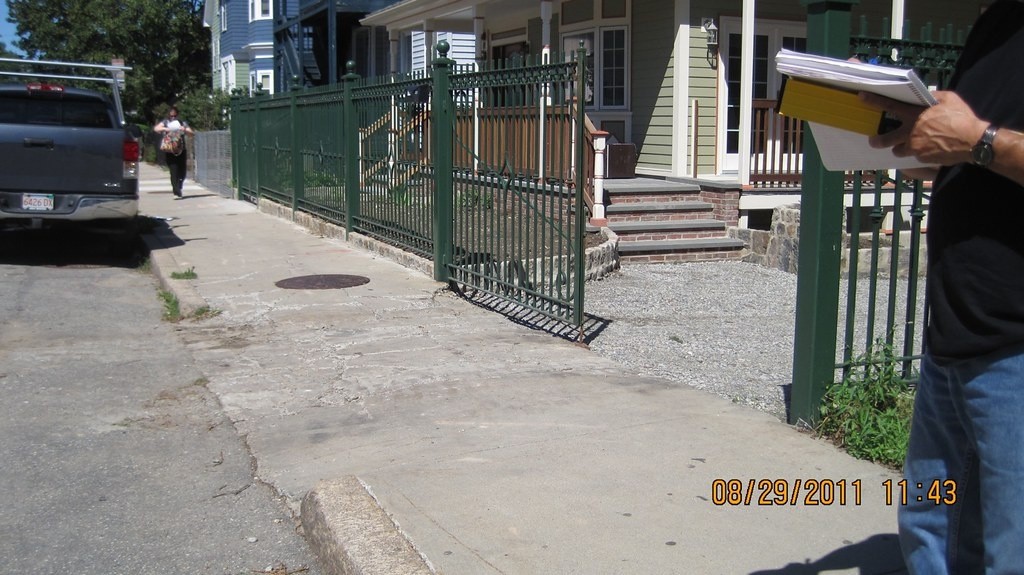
[774,47,945,169]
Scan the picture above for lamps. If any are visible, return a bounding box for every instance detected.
[706,19,719,46]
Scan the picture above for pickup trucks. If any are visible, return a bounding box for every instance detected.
[0,54,143,244]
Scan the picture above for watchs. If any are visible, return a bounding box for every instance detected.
[971,123,1001,170]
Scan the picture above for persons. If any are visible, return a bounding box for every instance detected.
[845,0,1024,575]
[154,106,195,200]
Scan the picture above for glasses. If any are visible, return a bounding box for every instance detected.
[171,116,176,117]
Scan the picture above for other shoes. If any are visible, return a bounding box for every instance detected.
[174,195,182,199]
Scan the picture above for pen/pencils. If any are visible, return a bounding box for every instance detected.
[870,55,881,65]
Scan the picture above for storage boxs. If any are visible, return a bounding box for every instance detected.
[603,142,637,179]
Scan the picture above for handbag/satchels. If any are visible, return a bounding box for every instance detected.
[161,130,184,156]
